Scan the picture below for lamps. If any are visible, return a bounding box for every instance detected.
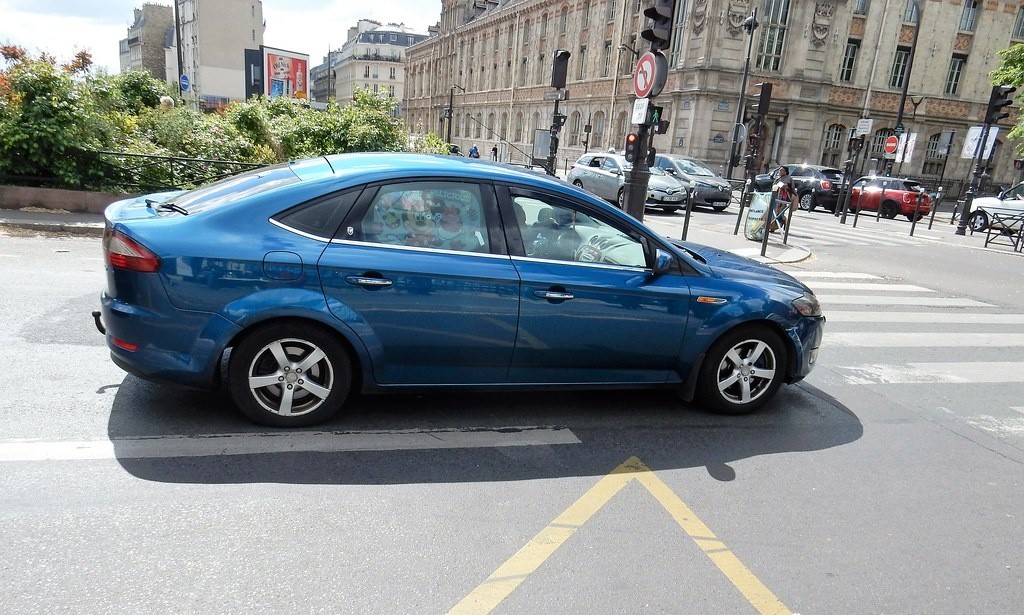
[618,44,640,59]
[452,84,464,92]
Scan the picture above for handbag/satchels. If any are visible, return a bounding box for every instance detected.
[787,189,798,211]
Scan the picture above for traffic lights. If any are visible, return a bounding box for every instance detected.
[625,133,639,162]
[552,115,569,127]
[551,50,573,89]
[646,105,665,125]
[987,84,1017,126]
[842,160,853,177]
[733,154,741,168]
[850,137,862,149]
[742,155,752,170]
[751,81,772,116]
[884,162,894,177]
[658,120,670,134]
[640,0,679,51]
[1013,158,1024,171]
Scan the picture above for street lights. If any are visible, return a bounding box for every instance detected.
[726,6,759,181]
[328,45,344,106]
[897,94,924,176]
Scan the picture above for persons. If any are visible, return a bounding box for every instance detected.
[469,145,478,157]
[513,202,544,256]
[492,144,497,162]
[769,166,795,232]
[161,96,174,112]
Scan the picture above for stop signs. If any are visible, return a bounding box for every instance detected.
[883,135,898,154]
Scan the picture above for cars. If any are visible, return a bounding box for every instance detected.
[644,151,733,213]
[447,143,462,157]
[90,151,827,431]
[567,151,688,214]
[968,180,1024,234]
[850,175,932,222]
[744,162,854,213]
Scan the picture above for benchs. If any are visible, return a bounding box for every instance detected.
[591,161,601,167]
